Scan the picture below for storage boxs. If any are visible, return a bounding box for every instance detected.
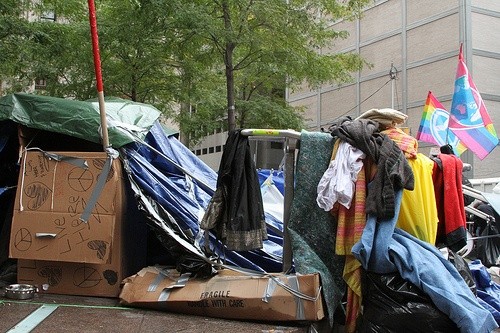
[9,152,328,320]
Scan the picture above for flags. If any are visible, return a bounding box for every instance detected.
[447,48,499,161]
[416,91,469,158]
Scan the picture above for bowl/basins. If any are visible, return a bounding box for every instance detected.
[5,284,36,300]
